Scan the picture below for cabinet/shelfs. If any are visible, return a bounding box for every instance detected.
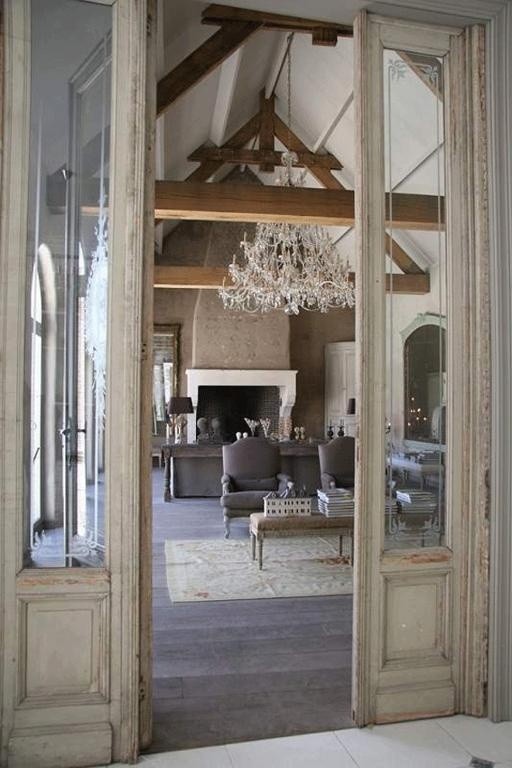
[325,340,358,439]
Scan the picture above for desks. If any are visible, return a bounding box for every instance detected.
[385,453,444,491]
[243,505,430,570]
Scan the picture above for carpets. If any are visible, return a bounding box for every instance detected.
[165,526,433,603]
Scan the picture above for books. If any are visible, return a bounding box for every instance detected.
[317,487,439,520]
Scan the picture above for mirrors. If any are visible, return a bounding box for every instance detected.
[403,324,446,444]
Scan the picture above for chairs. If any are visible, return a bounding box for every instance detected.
[218,435,297,539]
[316,436,399,553]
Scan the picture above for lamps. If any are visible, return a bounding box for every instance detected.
[216,28,364,316]
[166,395,194,444]
